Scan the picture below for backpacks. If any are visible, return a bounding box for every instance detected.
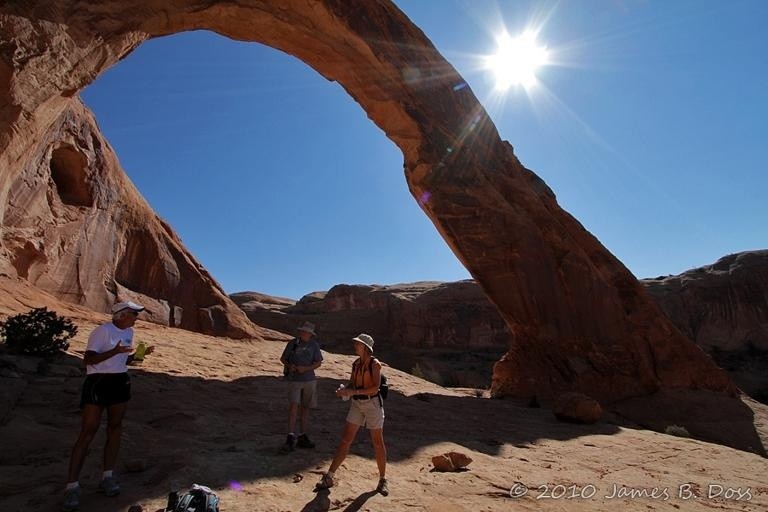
[351,357,389,400]
[165,483,219,511]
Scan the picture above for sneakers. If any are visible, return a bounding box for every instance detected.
[316,473,334,489]
[98,475,120,497]
[282,435,295,451]
[375,478,388,497]
[62,483,81,509]
[298,433,318,449]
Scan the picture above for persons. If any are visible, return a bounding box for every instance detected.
[64,300,154,508]
[280,321,324,451]
[315,334,390,495]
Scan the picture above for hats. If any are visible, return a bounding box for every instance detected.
[110,301,145,314]
[351,333,374,356]
[295,320,317,338]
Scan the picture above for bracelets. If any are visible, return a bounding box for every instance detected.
[357,389,360,395]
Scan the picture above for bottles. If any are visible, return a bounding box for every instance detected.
[135,340,147,361]
[339,383,350,402]
